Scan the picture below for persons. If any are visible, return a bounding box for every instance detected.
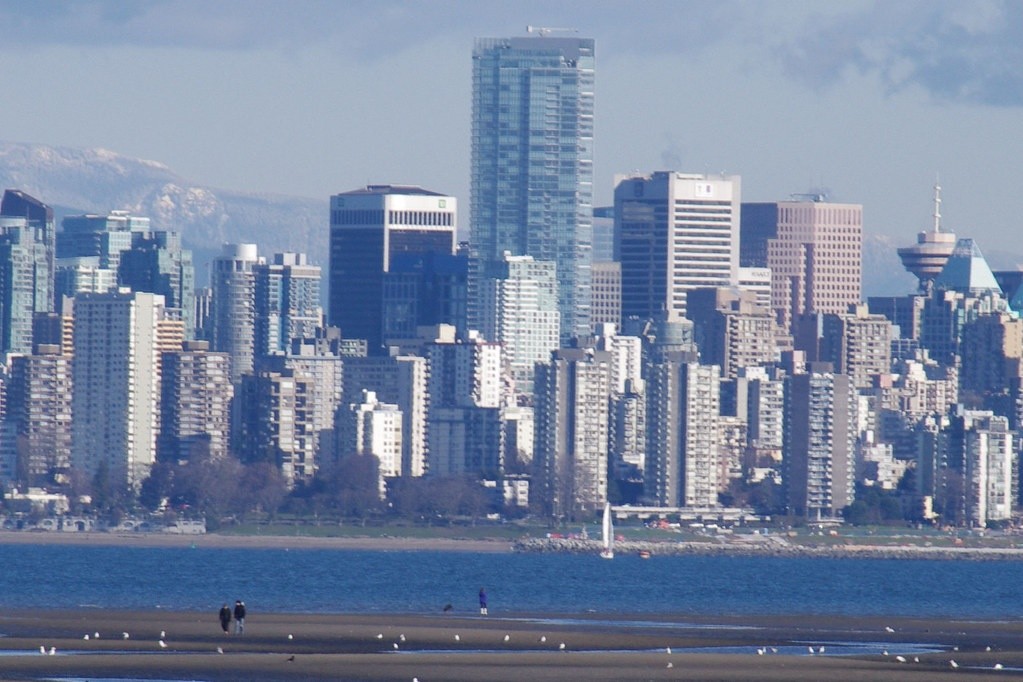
[479,588,488,614]
[220,605,232,635]
[234,601,246,636]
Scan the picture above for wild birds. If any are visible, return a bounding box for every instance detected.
[285,656,295,664]
[664,662,675,669]
[503,633,510,643]
[287,633,295,640]
[376,633,384,639]
[121,632,130,640]
[392,642,399,650]
[537,634,547,644]
[756,646,780,657]
[880,627,960,669]
[158,630,168,648]
[94,631,101,639]
[82,633,90,640]
[808,646,826,654]
[665,646,673,655]
[453,633,462,643]
[400,632,407,642]
[558,642,566,652]
[38,645,58,656]
[215,646,225,657]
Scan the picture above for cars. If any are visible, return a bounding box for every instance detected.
[0,517,207,535]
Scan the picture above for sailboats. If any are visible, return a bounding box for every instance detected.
[599,499,616,559]
